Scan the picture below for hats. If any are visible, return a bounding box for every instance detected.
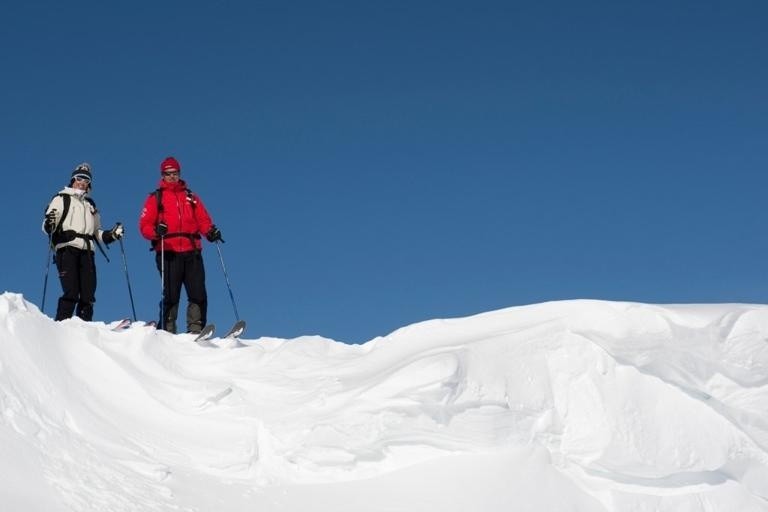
[72,163,92,184]
[161,157,180,171]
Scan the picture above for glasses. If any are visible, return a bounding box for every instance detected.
[74,177,90,185]
[163,171,179,175]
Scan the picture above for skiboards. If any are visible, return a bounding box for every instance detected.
[113,319,156,328]
[194,321,246,341]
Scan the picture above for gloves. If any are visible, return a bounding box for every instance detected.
[207,228,221,242]
[156,222,167,234]
[49,209,59,224]
[110,223,124,240]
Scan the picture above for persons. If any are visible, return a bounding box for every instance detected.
[41,162,124,321]
[137,155,221,334]
